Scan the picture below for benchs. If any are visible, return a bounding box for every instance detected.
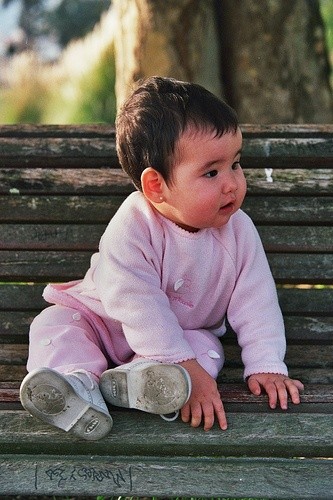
[1,123,333,500]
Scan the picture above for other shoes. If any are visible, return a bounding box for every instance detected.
[20,366,113,441]
[98,357,193,422]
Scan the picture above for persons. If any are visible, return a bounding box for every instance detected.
[18,75,306,441]
